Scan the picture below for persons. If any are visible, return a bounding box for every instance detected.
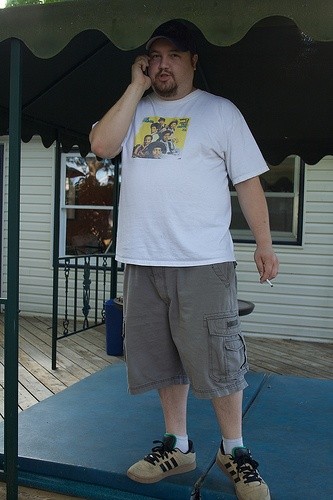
[73,199,107,254]
[89,31,279,500]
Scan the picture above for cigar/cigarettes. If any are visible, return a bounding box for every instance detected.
[266,279,273,287]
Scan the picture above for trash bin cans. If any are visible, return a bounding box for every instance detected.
[103,299,124,357]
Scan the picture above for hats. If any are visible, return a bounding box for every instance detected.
[140,21,204,56]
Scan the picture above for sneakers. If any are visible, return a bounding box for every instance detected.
[126,432,197,483]
[215,439,271,499]
